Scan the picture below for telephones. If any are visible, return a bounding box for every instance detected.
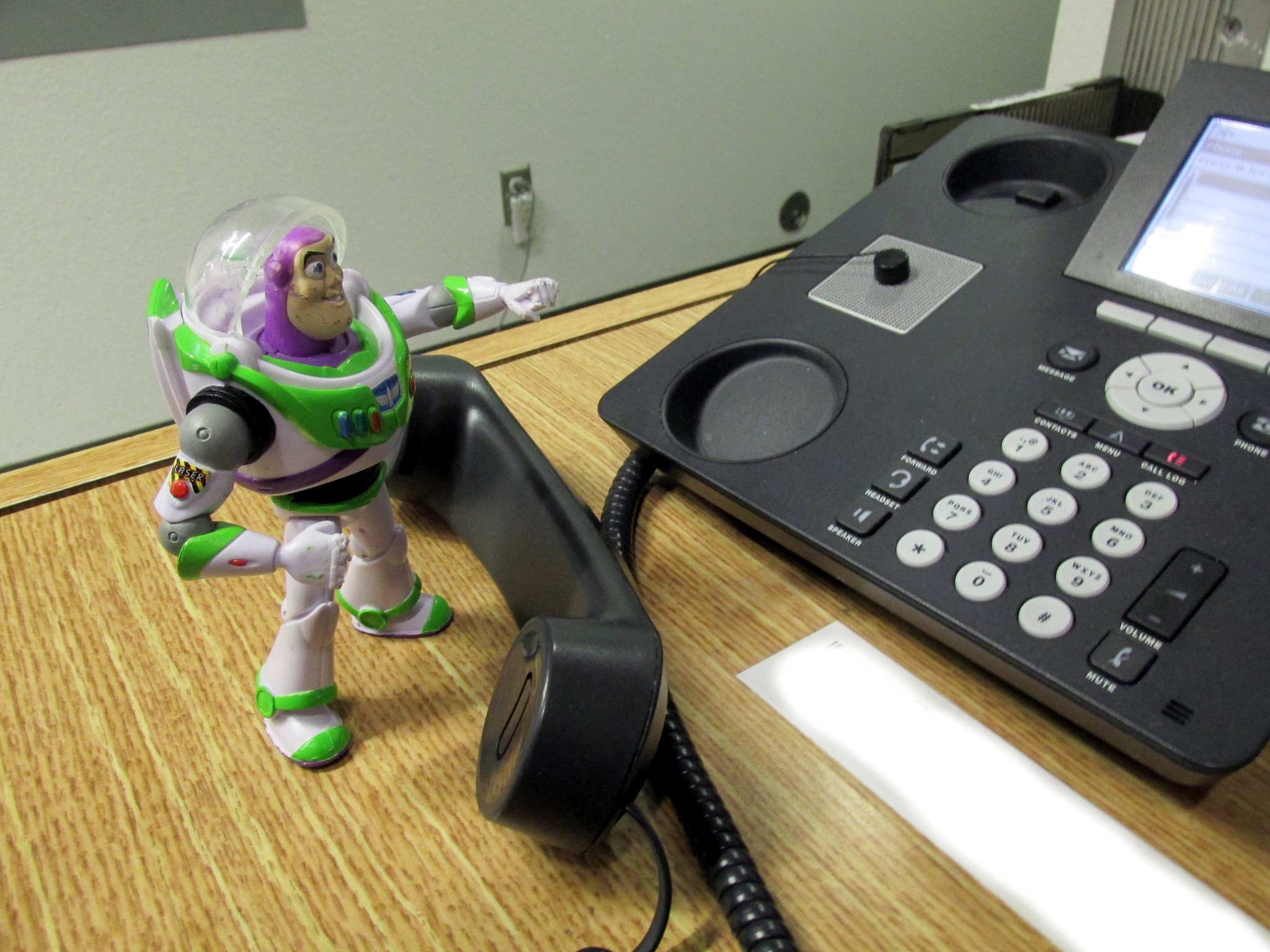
[379,56,1270,858]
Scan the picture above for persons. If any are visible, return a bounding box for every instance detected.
[144,190,558,772]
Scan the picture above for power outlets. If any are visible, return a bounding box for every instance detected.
[500,166,532,225]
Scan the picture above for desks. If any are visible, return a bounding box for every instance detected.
[3,250,1270,952]
